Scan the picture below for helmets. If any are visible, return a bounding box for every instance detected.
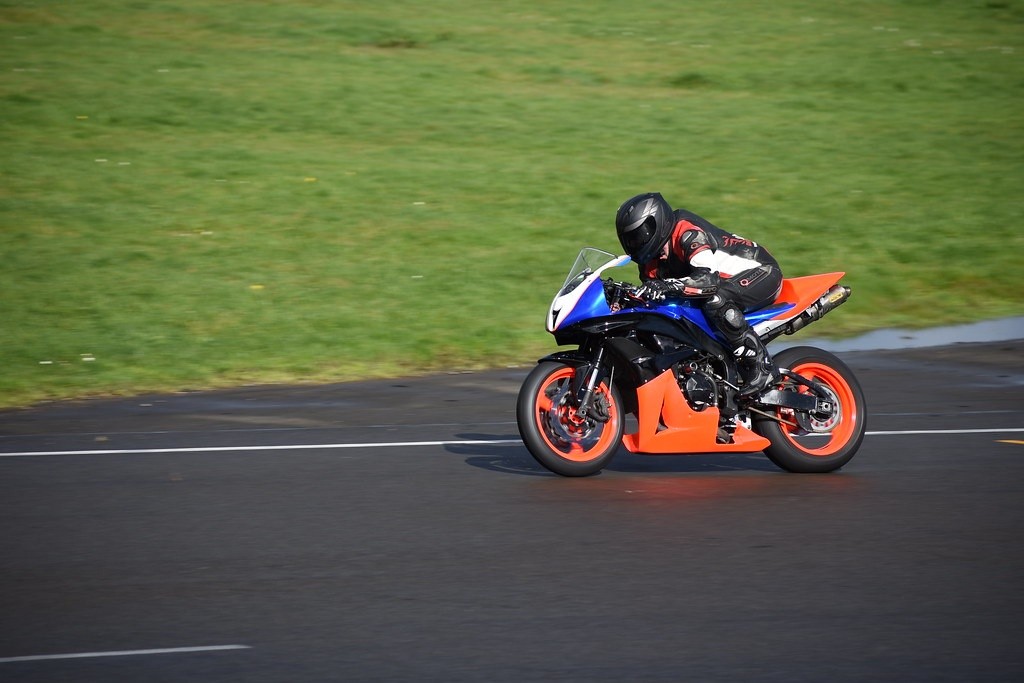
[616,192,675,262]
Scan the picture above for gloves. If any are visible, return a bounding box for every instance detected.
[640,281,686,305]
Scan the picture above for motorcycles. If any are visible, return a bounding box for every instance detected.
[516,247,868,478]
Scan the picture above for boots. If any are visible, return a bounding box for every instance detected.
[732,332,782,398]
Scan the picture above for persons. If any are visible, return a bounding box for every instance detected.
[616,192,782,403]
